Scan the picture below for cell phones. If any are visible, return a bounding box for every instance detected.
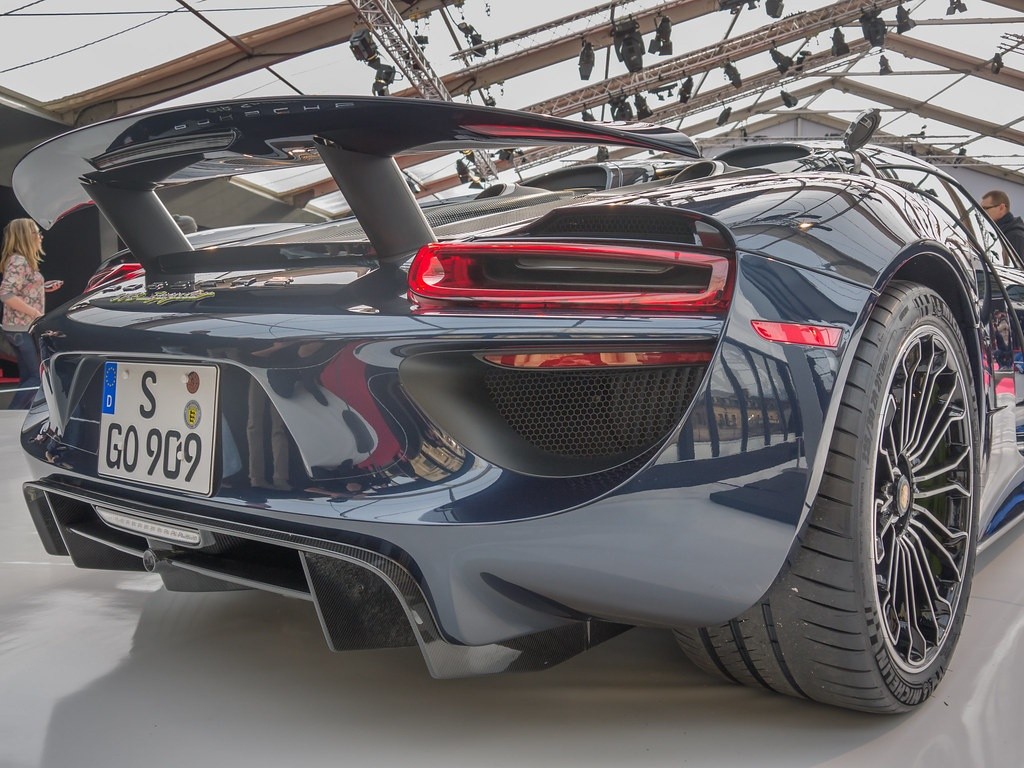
[44,281,62,288]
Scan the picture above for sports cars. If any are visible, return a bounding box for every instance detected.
[20,88,1022,718]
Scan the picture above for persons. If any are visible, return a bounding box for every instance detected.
[0,218,61,410]
[982,191,1024,263]
[991,311,1013,369]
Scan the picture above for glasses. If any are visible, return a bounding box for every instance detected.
[37,231,43,238]
[982,203,1008,210]
